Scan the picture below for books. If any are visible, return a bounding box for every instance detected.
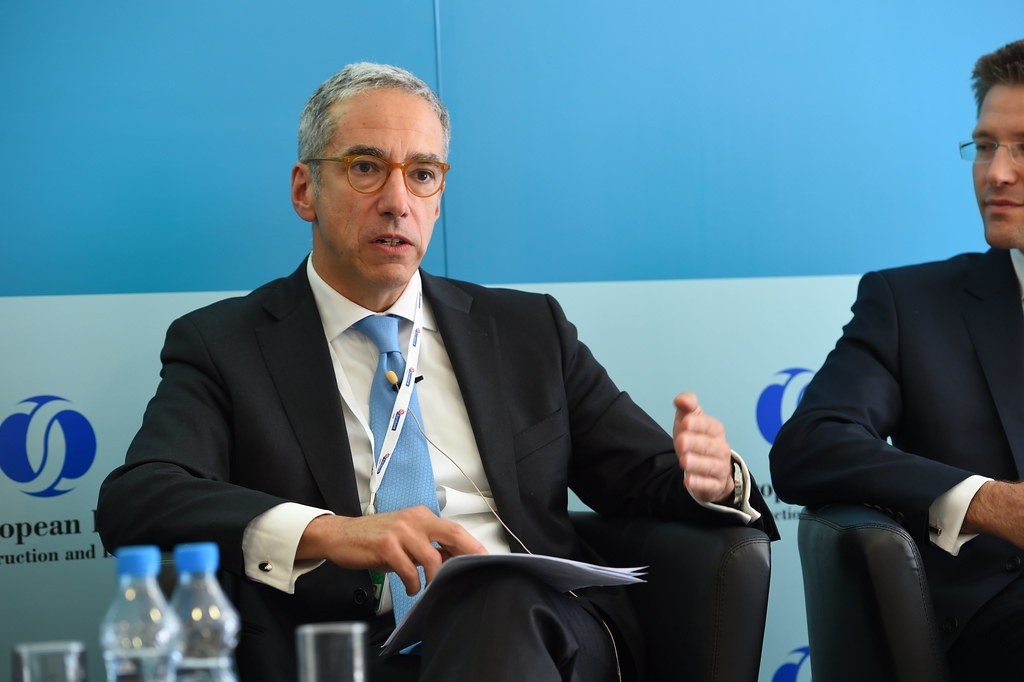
[378,552,650,660]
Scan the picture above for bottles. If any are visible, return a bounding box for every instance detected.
[168,542,240,682]
[100,546,181,682]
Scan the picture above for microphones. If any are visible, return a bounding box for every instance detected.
[385,370,399,385]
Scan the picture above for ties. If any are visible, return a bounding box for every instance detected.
[351,314,446,657]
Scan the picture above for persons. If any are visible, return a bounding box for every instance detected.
[767,38,1023,681]
[94,60,784,681]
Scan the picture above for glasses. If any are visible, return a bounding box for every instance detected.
[960,137,1024,167]
[302,153,449,198]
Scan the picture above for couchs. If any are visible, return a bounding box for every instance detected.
[798,503,952,682]
[160,513,772,682]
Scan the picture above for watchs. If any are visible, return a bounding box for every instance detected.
[733,457,745,509]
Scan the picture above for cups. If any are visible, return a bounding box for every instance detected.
[11,640,87,682]
[296,623,366,682]
